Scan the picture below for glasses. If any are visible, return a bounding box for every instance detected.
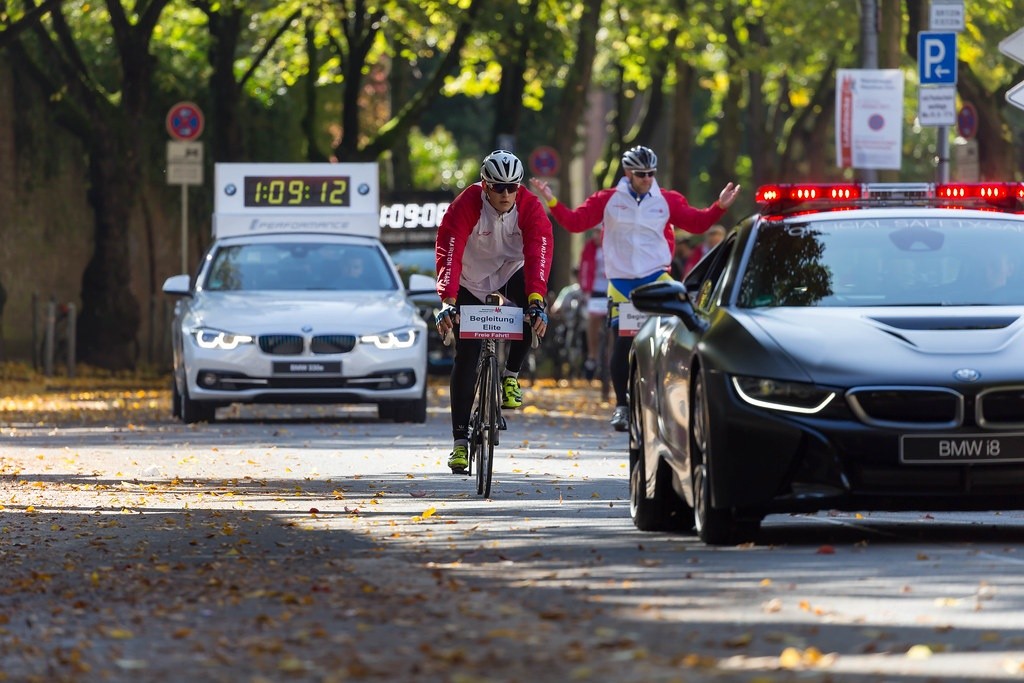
[628,170,659,178]
[485,181,520,193]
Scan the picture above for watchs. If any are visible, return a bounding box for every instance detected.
[529,300,546,310]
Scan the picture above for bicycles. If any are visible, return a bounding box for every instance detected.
[442,293,542,499]
[513,297,636,424]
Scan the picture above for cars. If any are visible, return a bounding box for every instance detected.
[162,230,437,425]
[383,225,457,378]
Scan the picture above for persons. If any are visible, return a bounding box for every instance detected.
[579,221,608,371]
[530,146,742,429]
[435,151,554,470]
[670,226,727,282]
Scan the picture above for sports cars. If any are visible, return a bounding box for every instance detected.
[625,182,1024,549]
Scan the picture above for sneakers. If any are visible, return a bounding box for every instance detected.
[611,405,630,430]
[448,444,468,469]
[502,376,523,409]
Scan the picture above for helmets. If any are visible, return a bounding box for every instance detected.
[621,145,658,168]
[481,149,523,184]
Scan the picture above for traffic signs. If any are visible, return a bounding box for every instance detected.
[917,28,958,86]
